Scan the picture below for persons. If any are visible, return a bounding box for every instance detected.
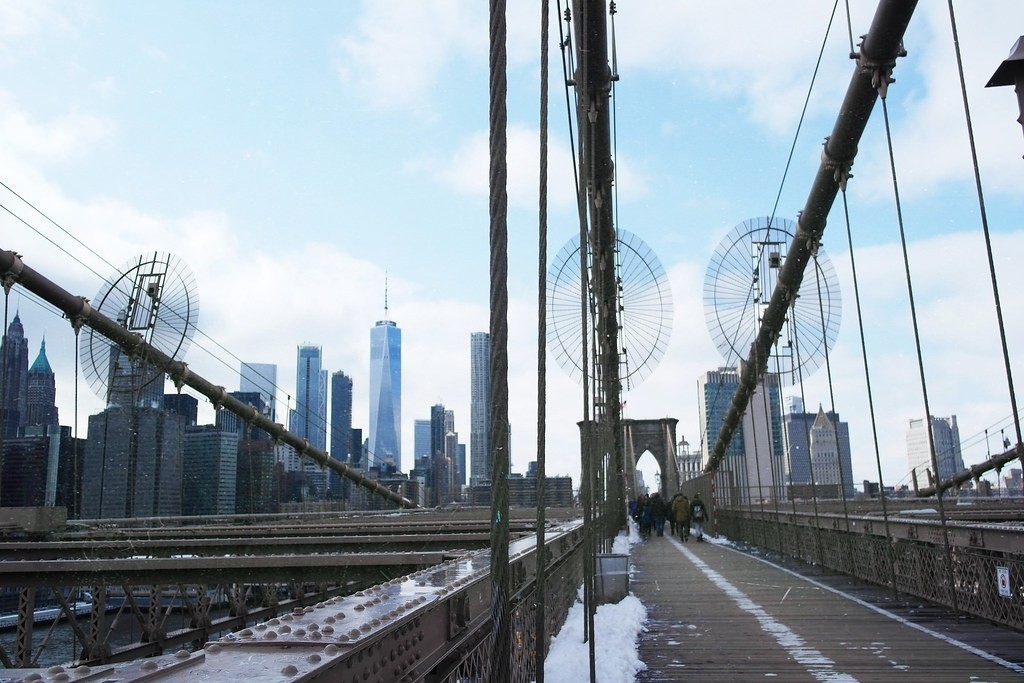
[671,492,691,542]
[627,493,679,540]
[689,493,709,542]
[683,496,692,536]
